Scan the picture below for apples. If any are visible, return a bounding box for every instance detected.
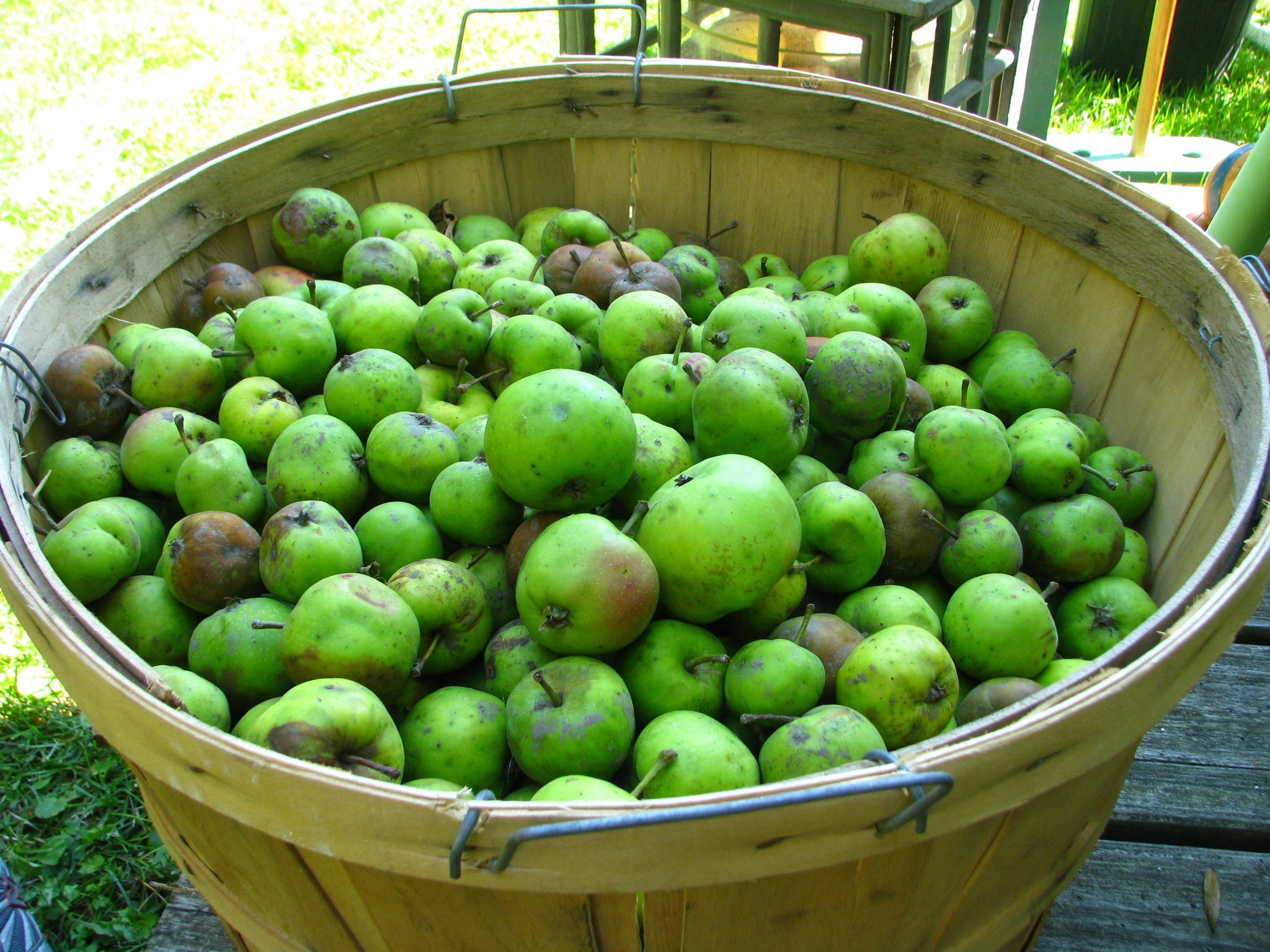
[39,186,1158,804]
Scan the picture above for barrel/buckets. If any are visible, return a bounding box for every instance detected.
[0,1,1270,952]
